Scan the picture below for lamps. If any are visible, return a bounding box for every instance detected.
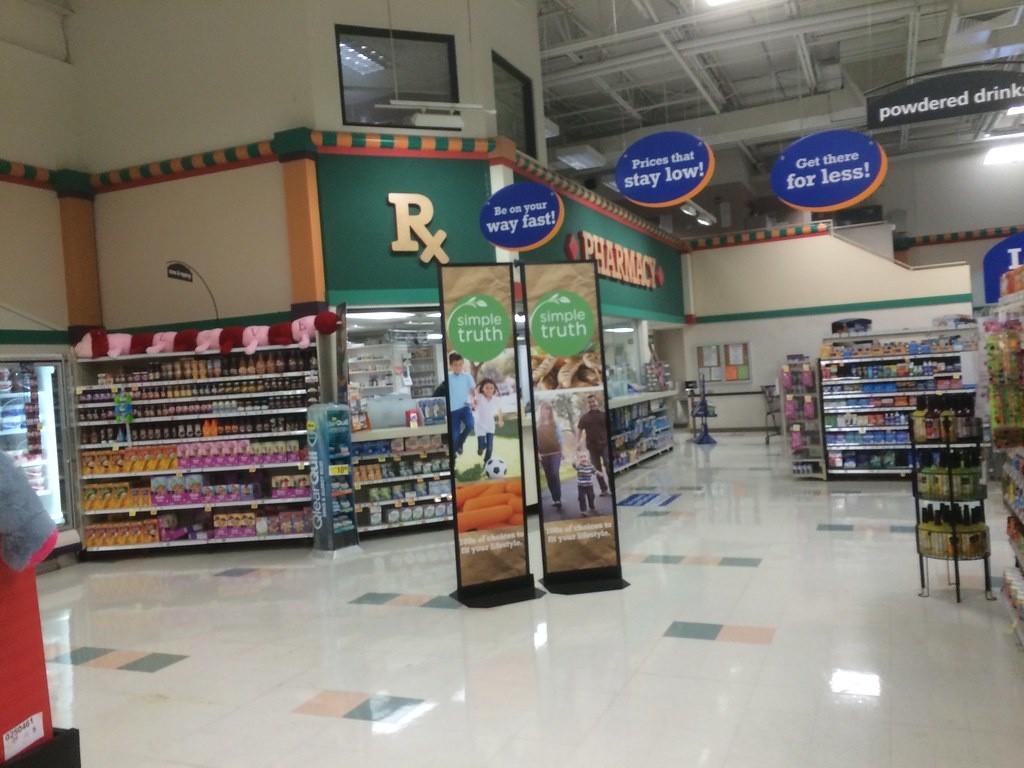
[679,204,697,216]
[697,214,711,226]
[389,0,482,127]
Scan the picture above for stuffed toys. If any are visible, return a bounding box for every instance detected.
[1,449,59,570]
[73,312,340,357]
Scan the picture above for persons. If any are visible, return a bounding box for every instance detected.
[571,447,605,516]
[468,378,504,462]
[575,394,612,497]
[449,353,476,462]
[536,402,565,507]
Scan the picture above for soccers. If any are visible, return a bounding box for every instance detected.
[484,457,508,480]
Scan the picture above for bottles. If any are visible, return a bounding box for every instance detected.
[923,360,936,375]
[148,350,317,379]
[885,412,901,425]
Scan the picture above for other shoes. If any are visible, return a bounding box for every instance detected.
[591,508,602,514]
[556,501,561,507]
[552,502,555,505]
[581,511,588,516]
[599,490,608,497]
[478,448,483,454]
[458,433,465,454]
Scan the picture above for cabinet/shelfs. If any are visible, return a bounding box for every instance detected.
[71,307,451,553]
[779,301,1024,641]
